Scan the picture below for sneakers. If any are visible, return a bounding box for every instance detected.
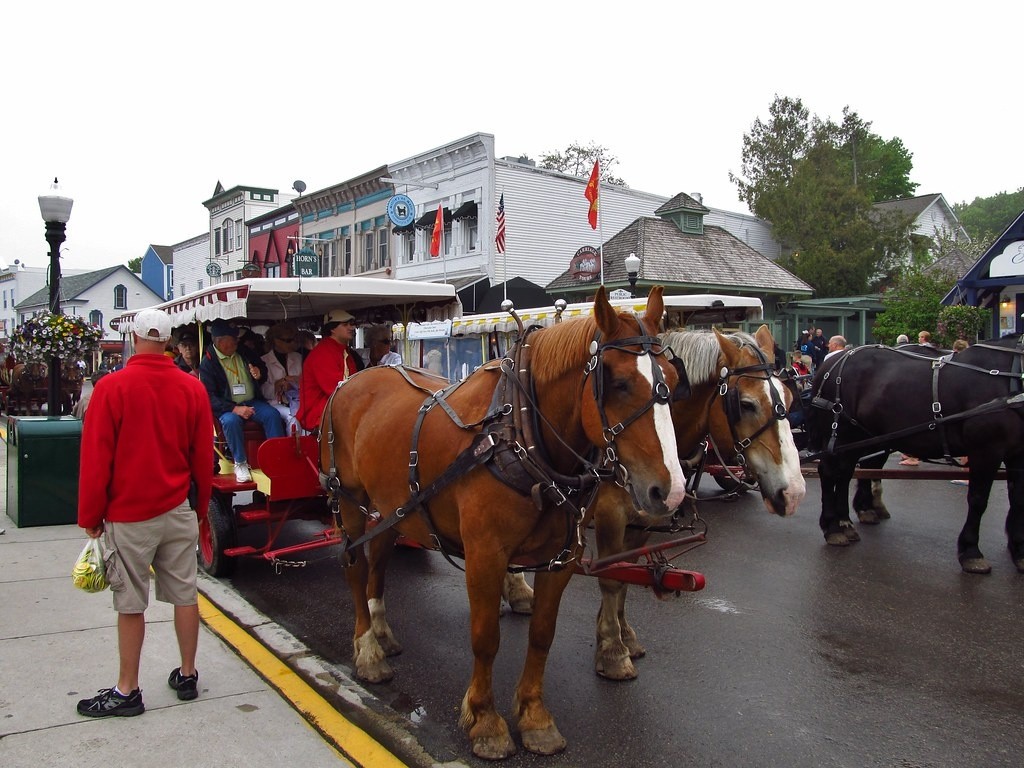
[168,666,199,701]
[77,685,146,717]
[234,460,252,482]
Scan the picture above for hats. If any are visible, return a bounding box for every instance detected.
[133,308,172,342]
[323,308,356,322]
[210,320,247,339]
[175,329,199,344]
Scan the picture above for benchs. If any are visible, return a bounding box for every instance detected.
[214,417,286,469]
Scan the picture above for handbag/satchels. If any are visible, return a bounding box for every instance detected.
[72,536,110,593]
[274,374,301,417]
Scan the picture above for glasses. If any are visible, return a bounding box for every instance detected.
[375,338,390,345]
[277,337,294,343]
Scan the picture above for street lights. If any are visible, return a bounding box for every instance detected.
[38,177,75,419]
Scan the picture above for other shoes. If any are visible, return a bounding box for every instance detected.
[899,454,918,466]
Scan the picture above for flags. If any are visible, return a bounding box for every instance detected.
[429,203,442,256]
[584,159,599,230]
[495,193,505,253]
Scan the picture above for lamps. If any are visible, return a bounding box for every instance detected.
[1000,295,1010,306]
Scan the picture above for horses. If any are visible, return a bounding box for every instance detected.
[807,333,1024,577]
[318,285,806,761]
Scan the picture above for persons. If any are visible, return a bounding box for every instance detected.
[174,310,402,484]
[77,309,214,716]
[791,324,847,389]
[897,331,969,485]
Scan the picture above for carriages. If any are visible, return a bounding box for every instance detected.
[391,294,1024,574]
[117,276,807,759]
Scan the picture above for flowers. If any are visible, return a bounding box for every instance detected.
[0,308,109,368]
